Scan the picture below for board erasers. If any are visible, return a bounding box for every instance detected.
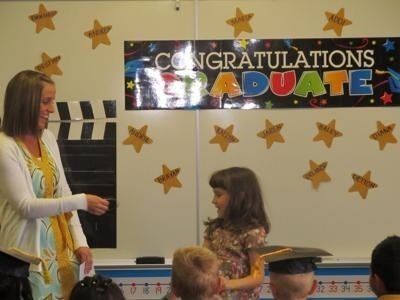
[136,257,164,264]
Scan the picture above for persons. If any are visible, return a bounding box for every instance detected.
[0,70,110,300]
[170,246,223,300]
[69,275,126,300]
[369,235,400,300]
[202,166,270,300]
[251,245,334,300]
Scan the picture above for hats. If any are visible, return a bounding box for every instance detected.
[252,245,335,276]
[0,248,41,279]
